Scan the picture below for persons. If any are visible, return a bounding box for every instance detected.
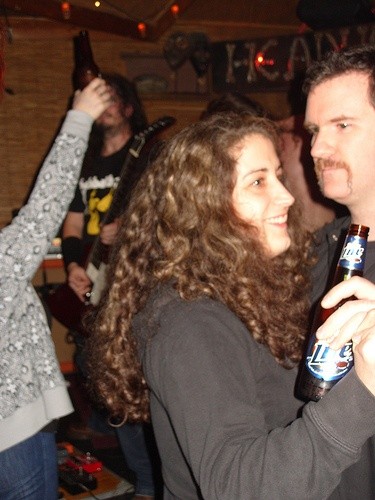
[0,77,114,500]
[304,46,375,307]
[200,91,353,311]
[60,72,165,500]
[82,113,375,500]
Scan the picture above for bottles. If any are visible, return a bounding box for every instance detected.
[74,29,102,130]
[295,224,370,401]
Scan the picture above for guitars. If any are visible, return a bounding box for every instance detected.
[47,116,177,338]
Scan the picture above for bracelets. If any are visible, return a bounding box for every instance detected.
[61,237,83,271]
